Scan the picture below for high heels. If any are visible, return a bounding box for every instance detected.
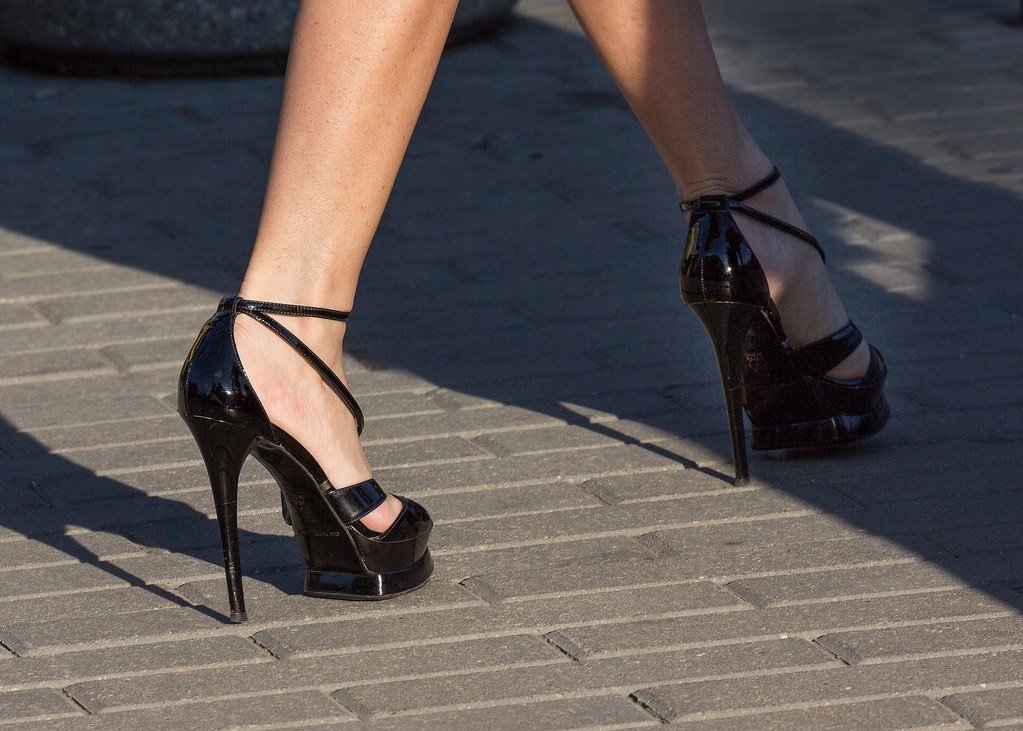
[678,165,892,486]
[174,296,433,623]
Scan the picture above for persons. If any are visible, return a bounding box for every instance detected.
[176,0,891,625]
